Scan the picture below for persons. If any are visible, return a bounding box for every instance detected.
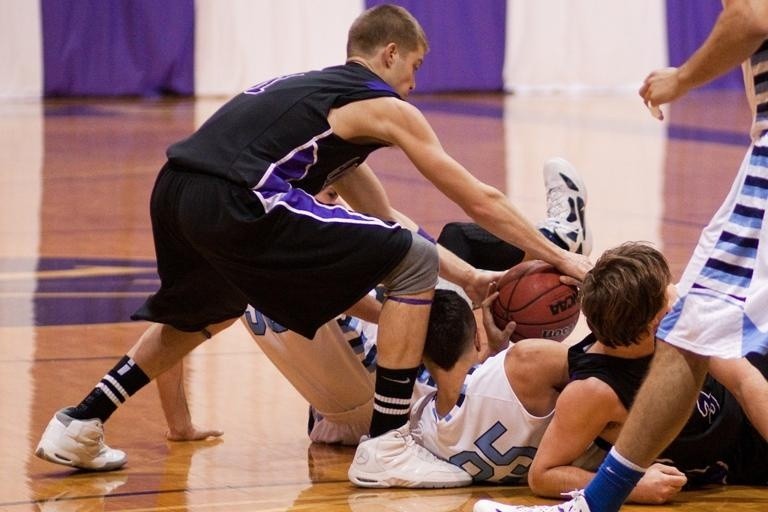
[528,242,768,506]
[156,157,594,446]
[473,0,768,512]
[409,274,585,484]
[34,4,597,489]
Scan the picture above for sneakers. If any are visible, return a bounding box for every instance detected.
[476,491,589,512]
[544,158,593,257]
[348,421,472,487]
[36,406,127,473]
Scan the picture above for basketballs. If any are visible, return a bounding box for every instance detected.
[491,259,581,343]
